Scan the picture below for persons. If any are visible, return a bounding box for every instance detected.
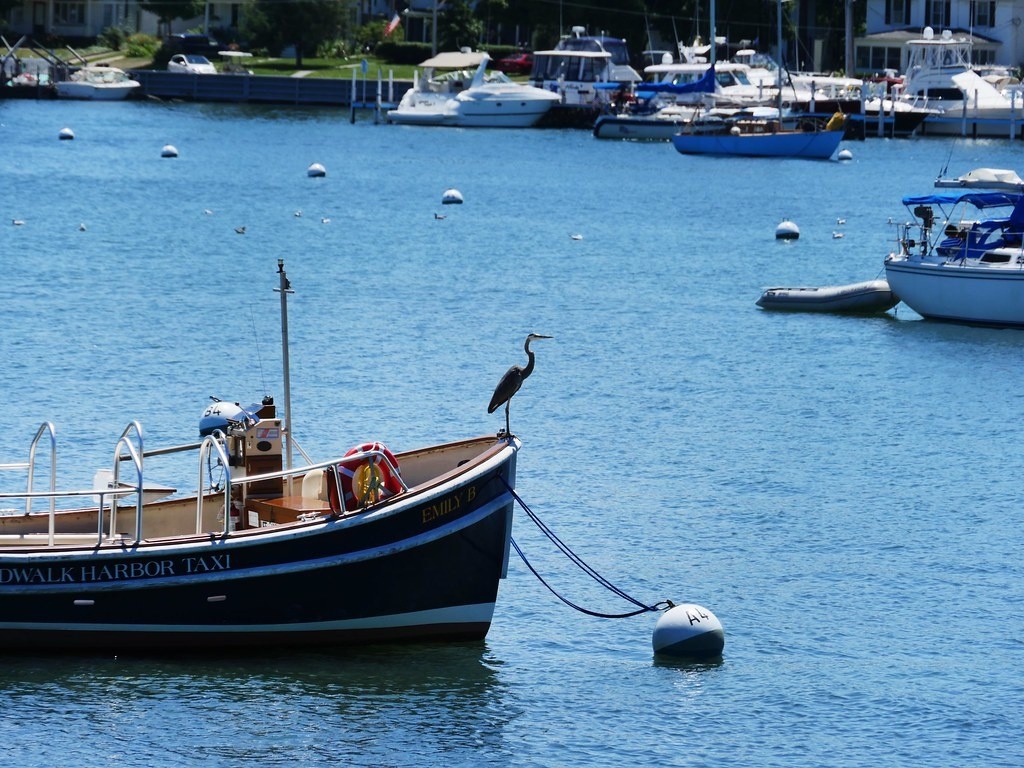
[940,225,966,255]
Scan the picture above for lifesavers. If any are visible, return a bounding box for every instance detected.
[327,441,405,515]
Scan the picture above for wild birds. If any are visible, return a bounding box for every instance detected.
[321,216,330,224]
[294,210,303,216]
[486,333,556,443]
[570,234,583,240]
[204,208,213,214]
[234,227,247,234]
[783,238,791,244]
[434,212,446,219]
[80,223,87,232]
[11,218,25,226]
[837,217,847,224]
[832,231,844,238]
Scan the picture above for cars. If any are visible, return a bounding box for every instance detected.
[161,31,233,60]
[498,51,535,76]
[167,53,217,74]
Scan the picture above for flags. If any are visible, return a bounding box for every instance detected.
[384,9,405,37]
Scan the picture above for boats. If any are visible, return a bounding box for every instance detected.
[387,45,564,129]
[0,256,525,648]
[754,280,903,315]
[882,129,1024,329]
[528,1,1024,162]
[54,64,142,100]
